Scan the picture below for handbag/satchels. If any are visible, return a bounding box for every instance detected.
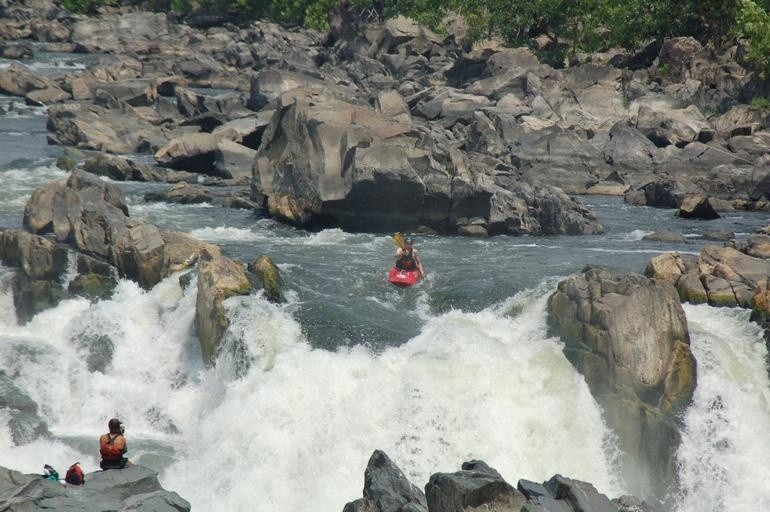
[65,463,84,486]
[41,465,58,481]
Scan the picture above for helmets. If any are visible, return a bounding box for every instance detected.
[405,237,413,245]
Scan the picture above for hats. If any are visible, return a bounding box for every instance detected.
[109,418,122,427]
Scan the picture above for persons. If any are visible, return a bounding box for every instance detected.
[100,418,128,469]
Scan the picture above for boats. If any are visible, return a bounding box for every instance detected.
[386,249,424,287]
